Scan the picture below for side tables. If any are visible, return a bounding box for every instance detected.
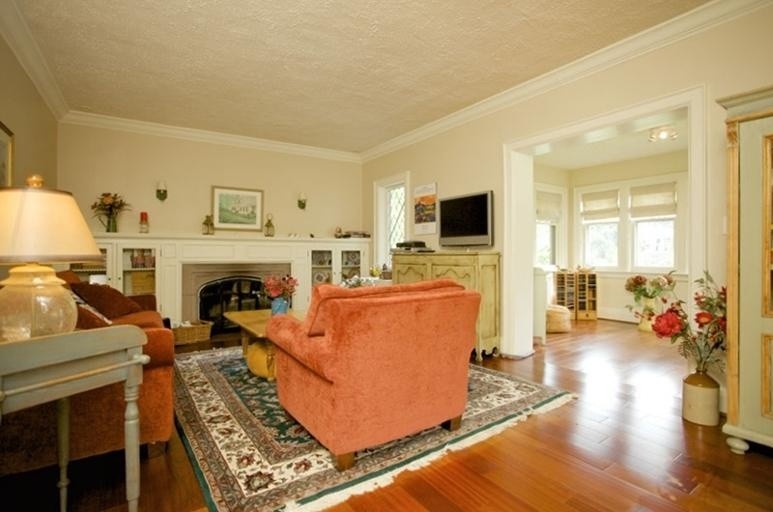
[0,324,151,512]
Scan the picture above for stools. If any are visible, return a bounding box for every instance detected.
[547,305,571,333]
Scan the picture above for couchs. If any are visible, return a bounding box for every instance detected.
[0,271,175,478]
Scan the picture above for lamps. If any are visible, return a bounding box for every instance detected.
[297,193,306,209]
[649,127,679,143]
[264,213,275,237]
[155,178,168,201]
[0,173,102,345]
[202,216,214,235]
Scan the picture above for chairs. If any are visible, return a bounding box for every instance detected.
[265,288,481,472]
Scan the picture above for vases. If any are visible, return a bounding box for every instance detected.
[682,368,720,427]
[637,296,656,332]
[99,212,117,232]
[271,298,289,316]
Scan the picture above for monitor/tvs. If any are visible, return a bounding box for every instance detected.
[437,190,493,247]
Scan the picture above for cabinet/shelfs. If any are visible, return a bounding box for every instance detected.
[391,250,502,363]
[65,239,156,297]
[311,247,361,289]
[576,272,598,320]
[555,271,576,320]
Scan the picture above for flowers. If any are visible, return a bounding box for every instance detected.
[261,273,300,298]
[624,275,673,298]
[90,191,136,220]
[633,271,727,370]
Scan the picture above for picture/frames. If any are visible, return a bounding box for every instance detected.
[0,121,15,188]
[211,185,264,232]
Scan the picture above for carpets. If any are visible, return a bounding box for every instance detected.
[174,344,580,512]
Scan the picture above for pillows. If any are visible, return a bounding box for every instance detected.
[303,278,465,337]
[76,303,113,329]
[70,281,144,319]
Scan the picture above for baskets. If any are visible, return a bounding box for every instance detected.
[172,320,214,345]
